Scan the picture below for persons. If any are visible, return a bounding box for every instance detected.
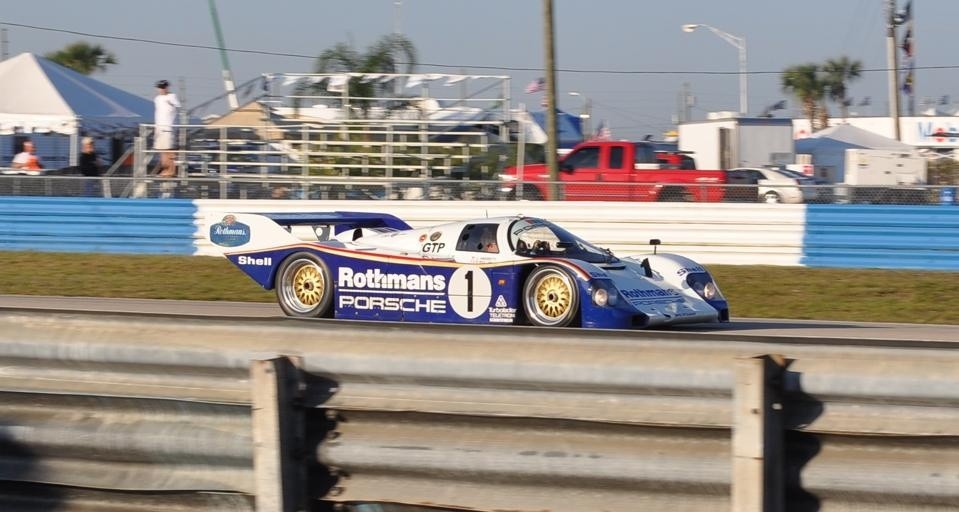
[11,141,41,171]
[152,78,182,177]
[77,136,103,177]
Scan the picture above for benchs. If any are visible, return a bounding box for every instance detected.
[132,93,514,202]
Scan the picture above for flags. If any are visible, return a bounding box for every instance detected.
[900,20,915,58]
[590,123,612,142]
[522,76,549,109]
[900,62,916,98]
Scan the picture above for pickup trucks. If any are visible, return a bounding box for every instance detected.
[500,140,759,202]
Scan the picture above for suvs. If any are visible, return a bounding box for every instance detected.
[656,150,695,169]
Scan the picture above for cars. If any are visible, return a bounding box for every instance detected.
[726,167,819,205]
[170,140,292,173]
[772,169,834,204]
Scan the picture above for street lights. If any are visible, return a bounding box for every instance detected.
[681,24,746,115]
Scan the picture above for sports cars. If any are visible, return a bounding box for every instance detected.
[209,210,730,327]
